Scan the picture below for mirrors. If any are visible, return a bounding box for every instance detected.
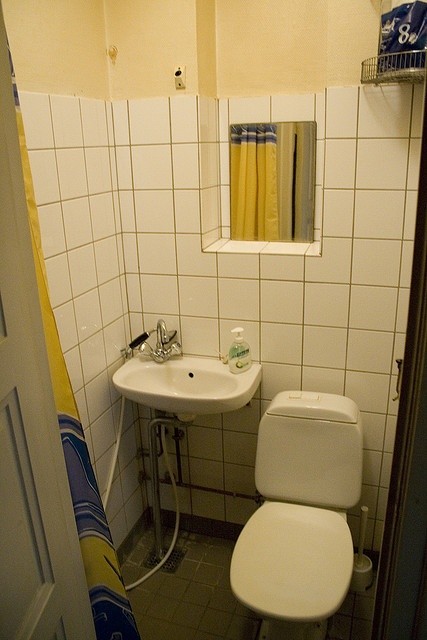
[229,121,317,243]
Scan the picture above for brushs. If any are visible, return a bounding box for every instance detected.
[354,506,371,571]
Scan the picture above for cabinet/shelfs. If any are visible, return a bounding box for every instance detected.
[361,49,426,85]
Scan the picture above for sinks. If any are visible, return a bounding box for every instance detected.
[111,350,262,400]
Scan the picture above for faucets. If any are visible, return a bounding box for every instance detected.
[153,319,171,363]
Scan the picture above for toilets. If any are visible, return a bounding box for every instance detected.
[230,390,365,640]
